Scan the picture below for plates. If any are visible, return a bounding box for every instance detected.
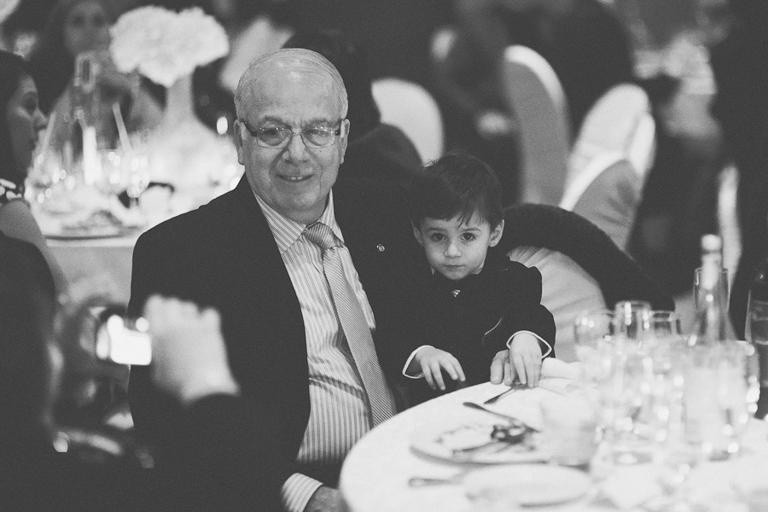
[409,417,554,462]
[460,462,595,507]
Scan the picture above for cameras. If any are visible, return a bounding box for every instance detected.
[89,310,160,368]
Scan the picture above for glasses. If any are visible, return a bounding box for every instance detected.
[242,121,340,148]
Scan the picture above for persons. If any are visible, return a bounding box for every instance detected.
[0,229,290,511]
[128,48,557,510]
[376,147,556,411]
[0,0,767,342]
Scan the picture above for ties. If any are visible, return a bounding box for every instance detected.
[305,223,392,426]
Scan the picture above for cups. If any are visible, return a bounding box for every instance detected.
[533,295,768,512]
